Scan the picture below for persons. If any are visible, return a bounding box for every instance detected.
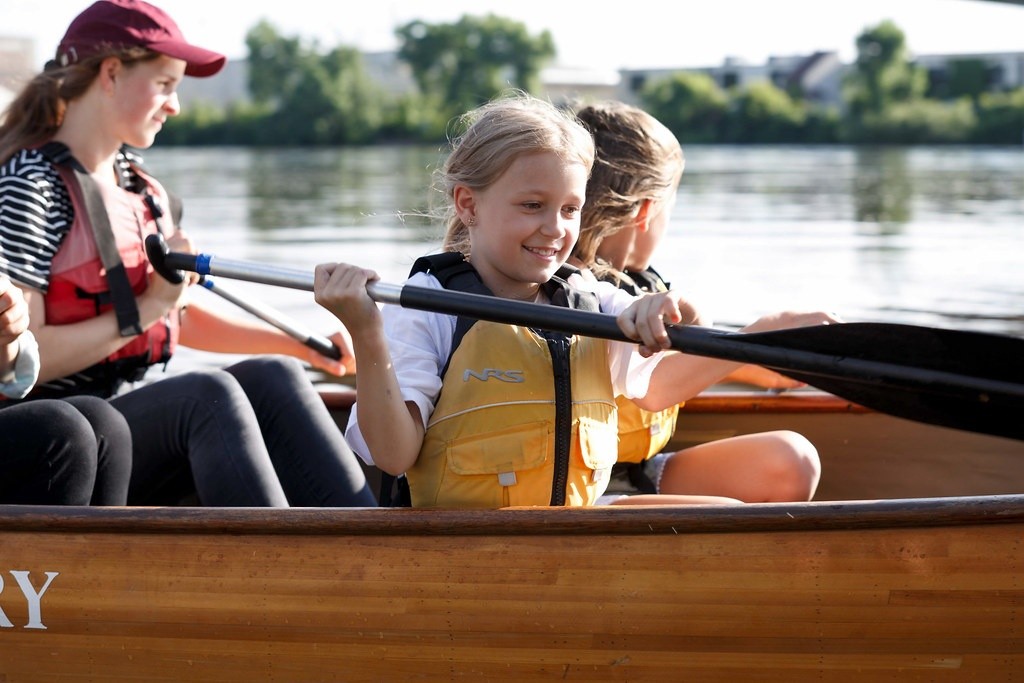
[0,0,383,508]
[572,103,842,500]
[0,280,133,505]
[311,98,745,508]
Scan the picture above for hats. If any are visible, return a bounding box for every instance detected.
[55,2,226,79]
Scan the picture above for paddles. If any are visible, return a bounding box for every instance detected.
[141,229,1024,446]
[194,271,344,363]
